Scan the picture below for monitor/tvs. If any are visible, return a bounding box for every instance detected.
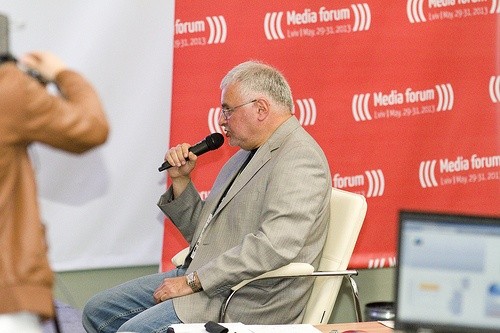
[392,208,500,333]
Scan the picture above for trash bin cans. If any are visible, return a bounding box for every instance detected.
[364,301,395,321]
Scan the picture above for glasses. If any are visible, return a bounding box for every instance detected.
[220,100,258,120]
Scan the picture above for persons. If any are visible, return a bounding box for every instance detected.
[0,43,109,333]
[81,61,332,333]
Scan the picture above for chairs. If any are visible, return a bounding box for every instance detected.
[170,186,367,324]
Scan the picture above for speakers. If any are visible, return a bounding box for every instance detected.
[158,132,224,172]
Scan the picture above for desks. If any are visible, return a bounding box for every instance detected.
[167,320,406,333]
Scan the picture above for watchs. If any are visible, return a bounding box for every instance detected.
[186,271,199,293]
